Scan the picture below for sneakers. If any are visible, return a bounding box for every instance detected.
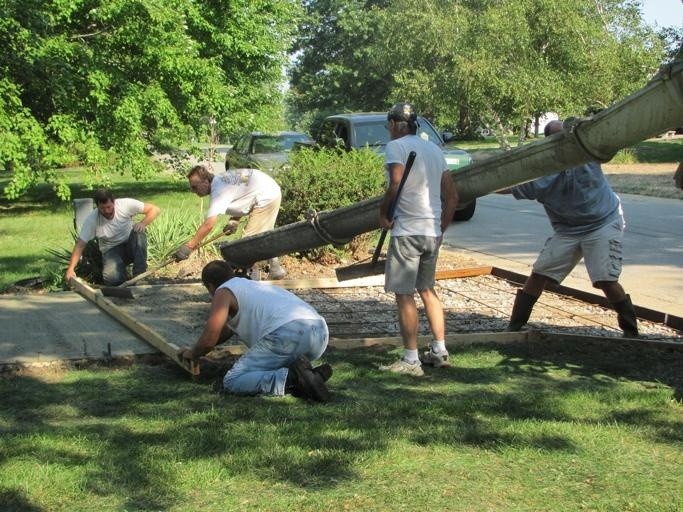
[265,266,287,281]
[133,273,148,287]
[379,360,425,377]
[315,363,333,383]
[420,352,451,369]
[247,270,261,282]
[287,354,330,404]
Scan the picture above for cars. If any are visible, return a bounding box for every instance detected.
[225,131,315,175]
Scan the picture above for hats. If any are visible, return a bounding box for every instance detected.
[388,102,417,124]
[202,261,234,281]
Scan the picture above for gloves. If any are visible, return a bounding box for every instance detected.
[223,219,239,234]
[172,247,191,261]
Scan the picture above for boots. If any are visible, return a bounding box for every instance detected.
[504,289,537,332]
[611,295,639,339]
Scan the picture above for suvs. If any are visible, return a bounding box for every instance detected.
[315,112,476,222]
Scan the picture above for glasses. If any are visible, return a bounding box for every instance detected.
[383,118,393,131]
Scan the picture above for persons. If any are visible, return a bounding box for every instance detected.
[174,165,287,279]
[63,188,161,285]
[494,119,643,339]
[179,260,332,403]
[376,103,460,378]
